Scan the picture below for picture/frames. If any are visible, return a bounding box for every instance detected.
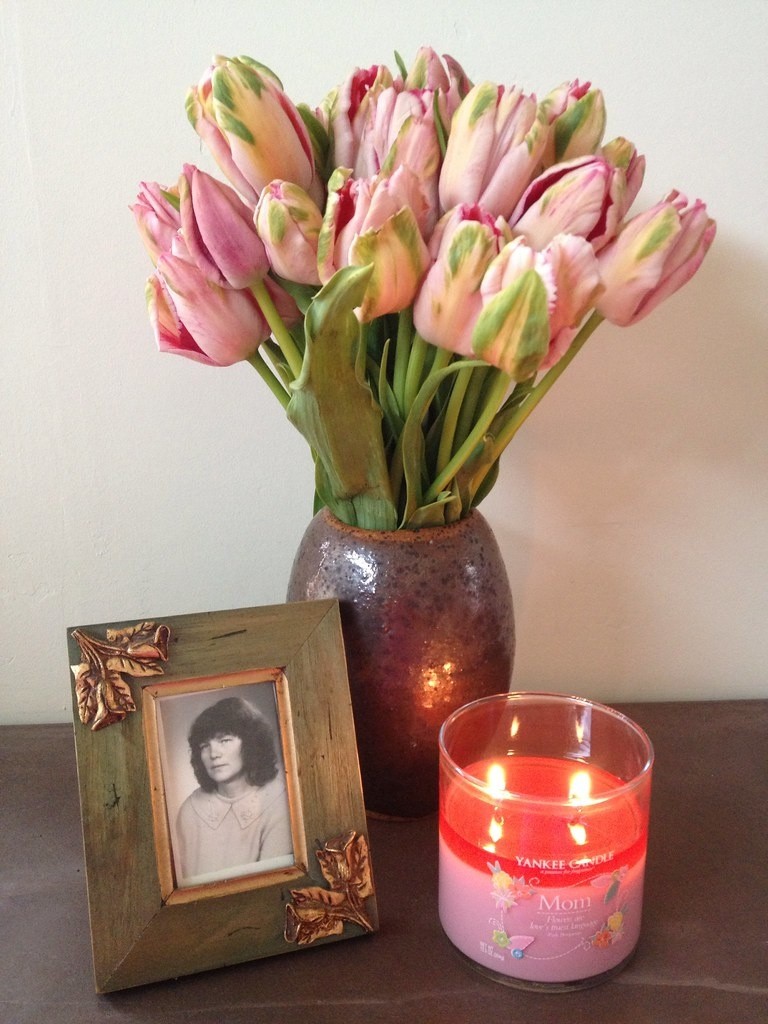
[67,597,379,995]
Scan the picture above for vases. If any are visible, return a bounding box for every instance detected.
[286,502,515,820]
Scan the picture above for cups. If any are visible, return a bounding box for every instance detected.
[434,689,653,992]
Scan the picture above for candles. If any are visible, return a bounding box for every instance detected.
[439,690,653,993]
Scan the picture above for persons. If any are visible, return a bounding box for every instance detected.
[175,696,291,880]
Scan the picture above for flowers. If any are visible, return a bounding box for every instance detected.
[124,46,715,529]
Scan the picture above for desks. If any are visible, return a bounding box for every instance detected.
[0,699,768,1024]
